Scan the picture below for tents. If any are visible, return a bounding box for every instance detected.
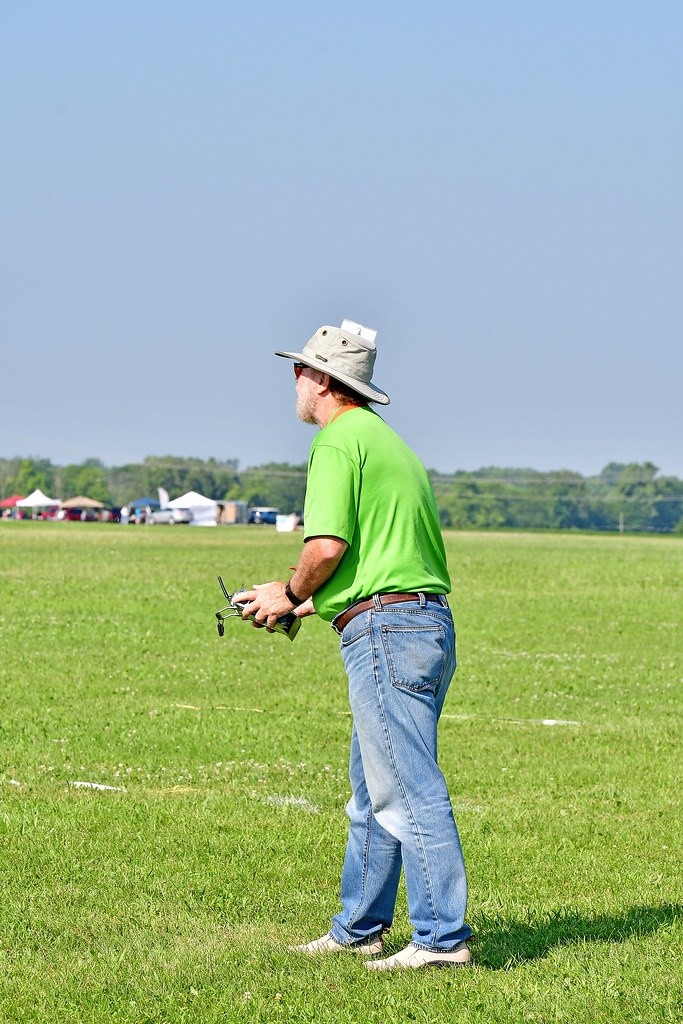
[1,488,105,518]
[127,496,160,509]
[158,487,218,526]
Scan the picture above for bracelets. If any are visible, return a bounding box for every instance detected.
[284,582,302,607]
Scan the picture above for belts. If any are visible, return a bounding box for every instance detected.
[332,593,439,635]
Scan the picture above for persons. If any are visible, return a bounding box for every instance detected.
[56,510,67,520]
[215,318,475,971]
[120,504,152,525]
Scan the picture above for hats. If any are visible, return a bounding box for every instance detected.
[275,326,391,405]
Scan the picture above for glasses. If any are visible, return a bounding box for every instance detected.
[293,362,336,392]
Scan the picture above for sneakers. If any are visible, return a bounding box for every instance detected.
[287,928,383,957]
[362,935,472,972]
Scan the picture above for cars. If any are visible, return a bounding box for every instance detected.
[149,506,195,527]
[37,504,151,525]
[246,506,281,525]
